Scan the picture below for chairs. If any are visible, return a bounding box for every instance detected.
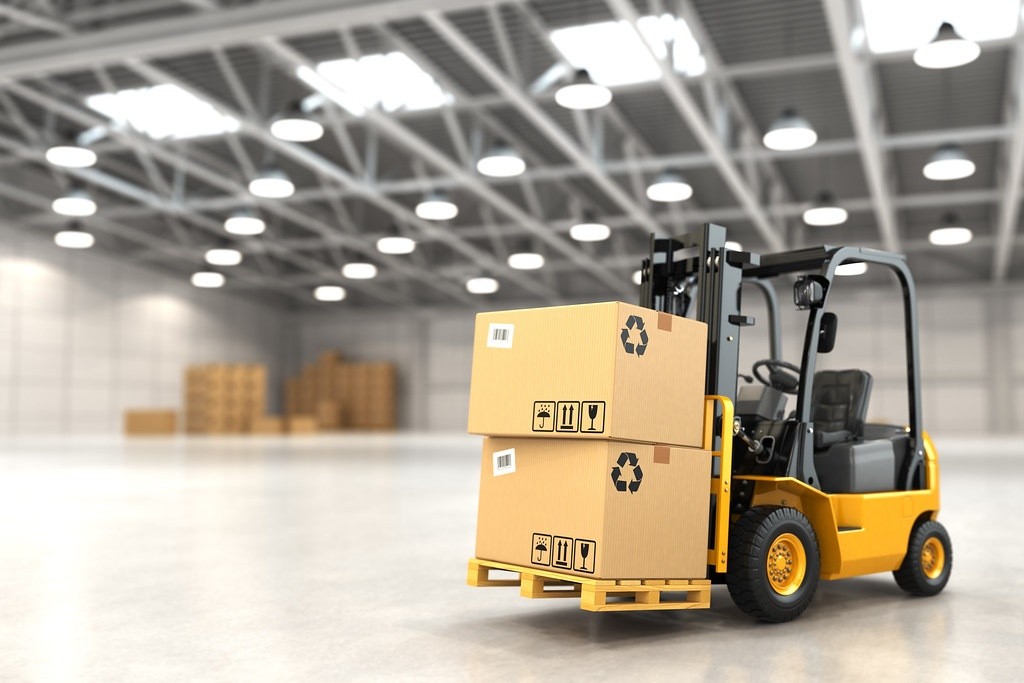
[811,369,872,449]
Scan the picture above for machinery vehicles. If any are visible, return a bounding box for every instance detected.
[466,220,952,626]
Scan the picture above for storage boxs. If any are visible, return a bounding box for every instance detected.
[468,301,709,450]
[124,353,395,436]
[476,435,711,580]
